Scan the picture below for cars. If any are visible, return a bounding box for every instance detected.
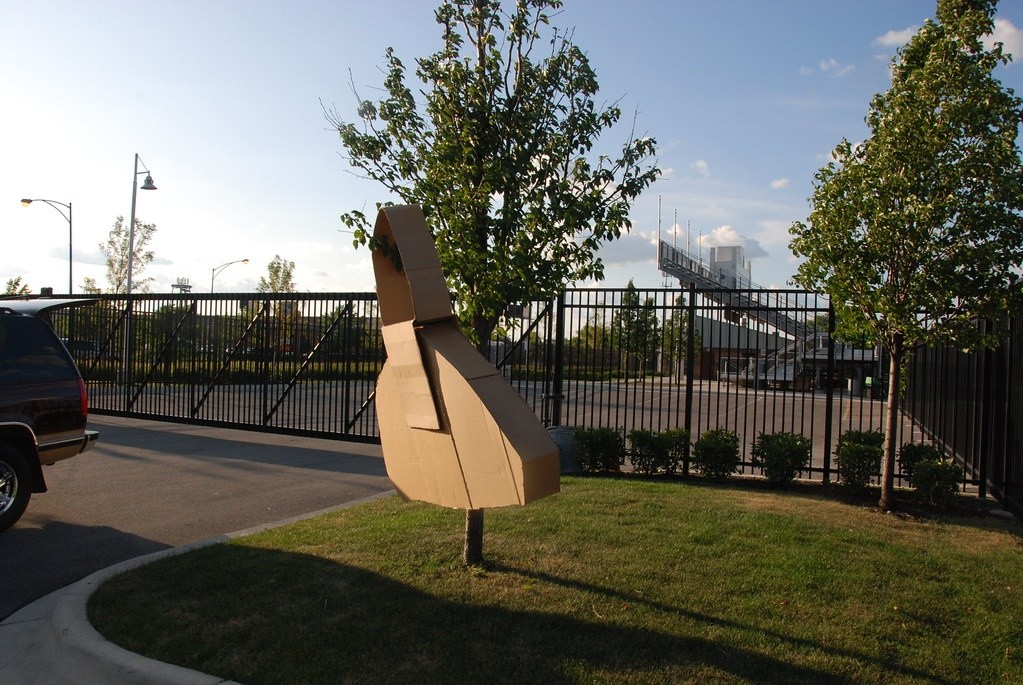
[200,344,214,352]
[225,348,234,354]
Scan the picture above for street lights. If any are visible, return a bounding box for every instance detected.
[122,152,157,379]
[22,197,74,356]
[211,259,249,292]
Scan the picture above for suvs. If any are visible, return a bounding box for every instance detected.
[0,306,102,533]
